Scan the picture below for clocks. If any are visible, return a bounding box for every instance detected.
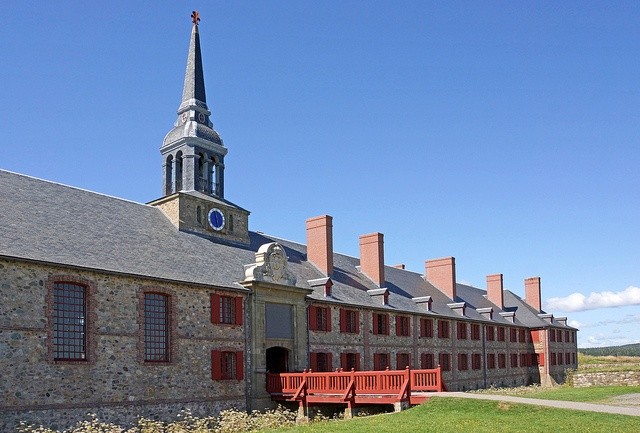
[205,203,228,234]
